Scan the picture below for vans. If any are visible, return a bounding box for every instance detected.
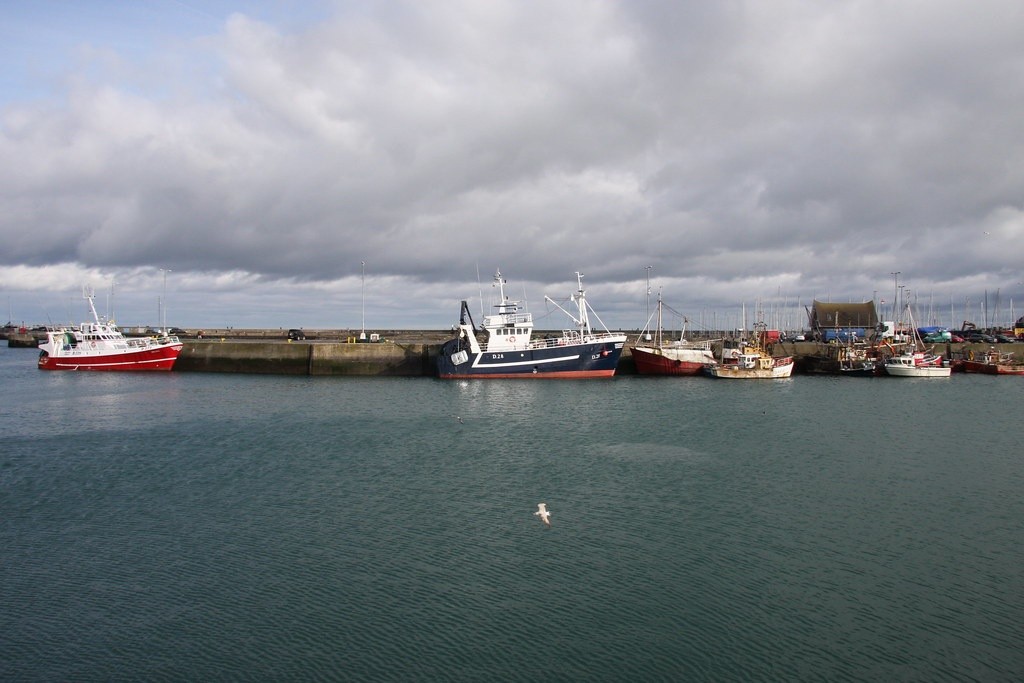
[822,328,856,344]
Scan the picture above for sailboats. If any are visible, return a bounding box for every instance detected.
[805,285,1024,378]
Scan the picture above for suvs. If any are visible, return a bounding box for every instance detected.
[287,328,305,341]
[970,334,994,344]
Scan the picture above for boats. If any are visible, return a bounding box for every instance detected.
[7,326,39,348]
[703,300,795,379]
[0,321,17,340]
[38,285,184,373]
[434,261,629,380]
[630,285,718,378]
[29,326,49,341]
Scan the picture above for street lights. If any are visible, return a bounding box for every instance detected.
[898,285,906,331]
[359,260,368,343]
[160,268,172,334]
[644,265,654,341]
[890,272,901,326]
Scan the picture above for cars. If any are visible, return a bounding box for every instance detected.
[796,335,805,341]
[952,335,964,344]
[170,328,186,333]
[995,335,1015,344]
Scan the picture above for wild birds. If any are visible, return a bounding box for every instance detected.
[533,504,551,524]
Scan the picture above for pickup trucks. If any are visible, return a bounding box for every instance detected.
[922,332,952,344]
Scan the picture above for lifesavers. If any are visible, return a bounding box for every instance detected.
[509,336,516,343]
[91,342,96,348]
[732,352,738,358]
